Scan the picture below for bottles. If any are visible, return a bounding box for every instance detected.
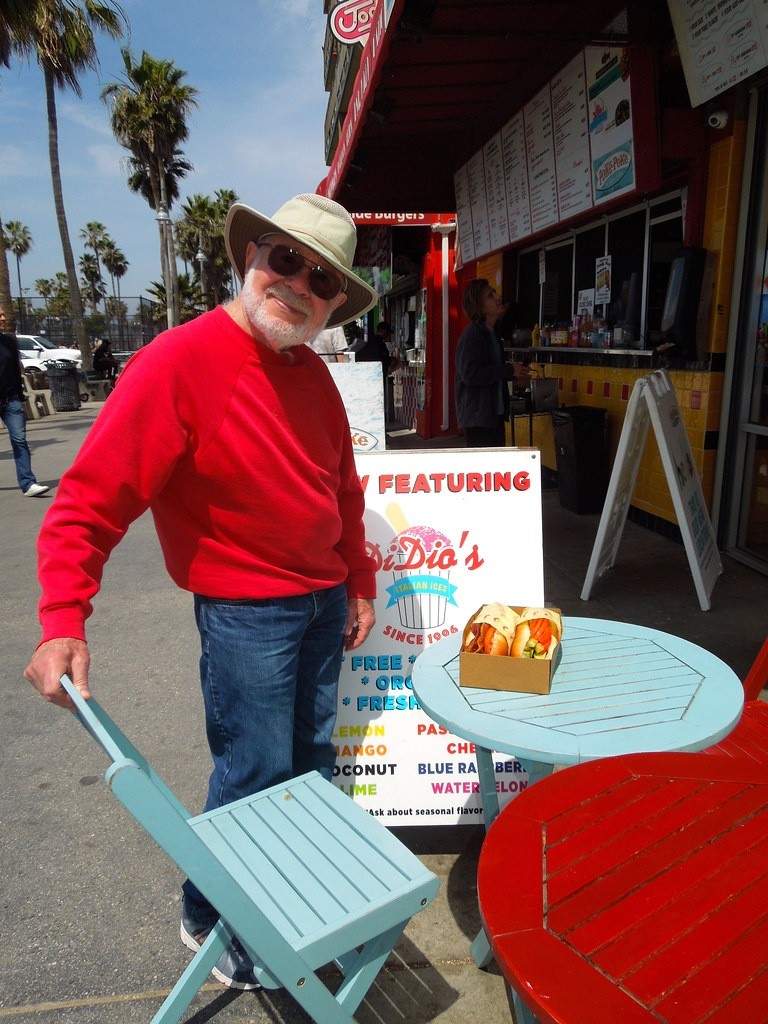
[532,324,540,347]
[544,333,551,345]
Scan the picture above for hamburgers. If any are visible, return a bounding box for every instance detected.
[462,622,510,656]
[510,618,558,660]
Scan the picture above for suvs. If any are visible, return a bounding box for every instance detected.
[16,334,83,369]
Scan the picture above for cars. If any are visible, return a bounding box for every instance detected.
[16,350,58,375]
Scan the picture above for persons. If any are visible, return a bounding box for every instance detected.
[0,305,49,498]
[69,337,119,380]
[356,321,396,380]
[24,191,381,989]
[457,278,532,448]
[346,326,367,351]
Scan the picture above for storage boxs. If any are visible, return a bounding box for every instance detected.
[460,604,562,694]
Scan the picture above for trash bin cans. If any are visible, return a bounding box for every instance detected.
[553,405,608,515]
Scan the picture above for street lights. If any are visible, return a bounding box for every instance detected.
[155,206,173,329]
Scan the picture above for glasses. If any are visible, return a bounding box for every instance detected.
[257,243,345,301]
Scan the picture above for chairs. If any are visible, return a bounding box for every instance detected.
[58,674,440,1024]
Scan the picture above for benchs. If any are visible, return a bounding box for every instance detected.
[83,369,117,401]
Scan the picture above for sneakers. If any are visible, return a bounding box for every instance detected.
[24,484,50,497]
[179,895,261,989]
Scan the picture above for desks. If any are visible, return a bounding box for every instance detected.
[476,750,768,1024]
[413,616,745,969]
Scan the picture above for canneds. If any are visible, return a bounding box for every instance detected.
[603,330,613,349]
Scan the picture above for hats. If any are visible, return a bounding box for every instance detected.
[224,194,379,330]
[377,322,395,334]
